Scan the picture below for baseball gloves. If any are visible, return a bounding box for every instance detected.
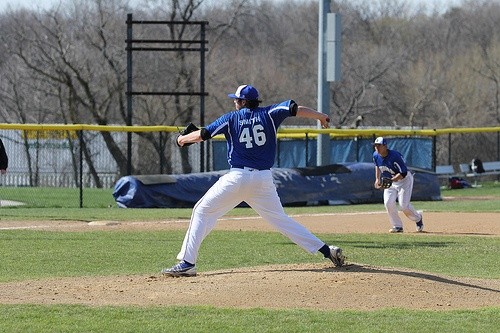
[176,123,200,148]
[379,172,393,189]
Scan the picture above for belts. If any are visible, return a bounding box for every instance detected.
[233,166,270,171]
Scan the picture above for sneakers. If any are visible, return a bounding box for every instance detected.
[329,246,345,267]
[162,259,197,277]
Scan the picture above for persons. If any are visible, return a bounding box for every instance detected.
[0,139,8,174]
[371,137,423,233]
[162,85,345,276]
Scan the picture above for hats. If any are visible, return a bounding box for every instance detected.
[228,85,262,103]
[371,137,387,144]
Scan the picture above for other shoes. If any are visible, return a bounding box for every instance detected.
[389,227,404,233]
[415,210,424,232]
